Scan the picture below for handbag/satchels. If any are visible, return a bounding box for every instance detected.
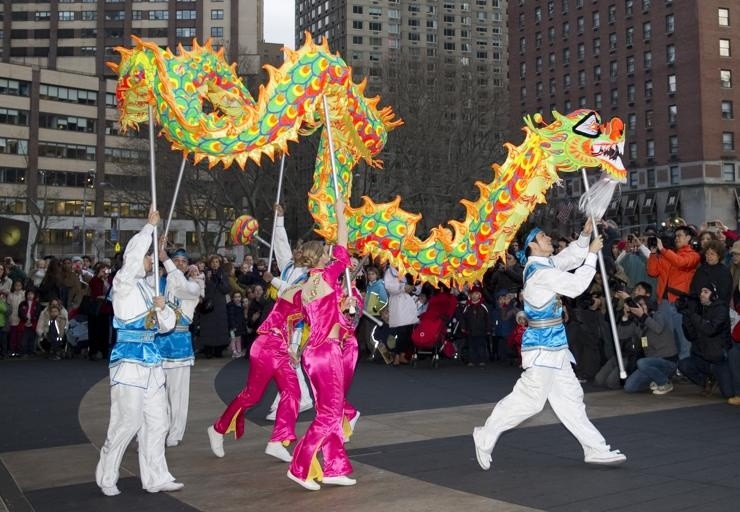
[195,273,221,314]
[78,285,112,317]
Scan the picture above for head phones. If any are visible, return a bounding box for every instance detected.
[709,282,719,302]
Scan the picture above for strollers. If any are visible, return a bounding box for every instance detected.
[61,315,90,358]
[409,292,470,368]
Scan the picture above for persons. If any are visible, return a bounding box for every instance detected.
[135,234,200,447]
[473,216,626,470]
[0,252,123,362]
[263,203,314,420]
[552,220,740,406]
[192,253,279,359]
[206,286,307,463]
[95,202,184,496]
[285,199,358,491]
[357,241,527,372]
[338,254,364,444]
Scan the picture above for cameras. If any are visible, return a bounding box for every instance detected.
[608,278,633,295]
[6,258,11,266]
[692,240,699,251]
[625,298,638,313]
[648,219,680,247]
[627,229,640,242]
[103,269,111,273]
[578,291,602,311]
[665,287,702,312]
[707,222,715,227]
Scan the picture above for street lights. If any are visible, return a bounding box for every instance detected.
[82,169,96,255]
[101,183,120,242]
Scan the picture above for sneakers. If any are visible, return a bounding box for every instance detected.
[300,400,312,413]
[146,480,184,493]
[650,381,658,390]
[704,376,718,397]
[208,425,225,457]
[730,397,739,404]
[349,410,360,431]
[168,438,178,447]
[287,470,321,491]
[322,475,357,486]
[653,382,673,395]
[472,425,493,470]
[343,437,350,442]
[584,449,628,465]
[267,442,293,463]
[102,482,121,495]
[266,411,277,421]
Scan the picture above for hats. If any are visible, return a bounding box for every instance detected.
[470,286,479,292]
[496,287,509,299]
[73,256,83,263]
[729,241,740,255]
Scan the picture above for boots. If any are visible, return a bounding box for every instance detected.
[400,353,407,363]
[236,336,246,357]
[393,354,399,365]
[230,337,241,358]
